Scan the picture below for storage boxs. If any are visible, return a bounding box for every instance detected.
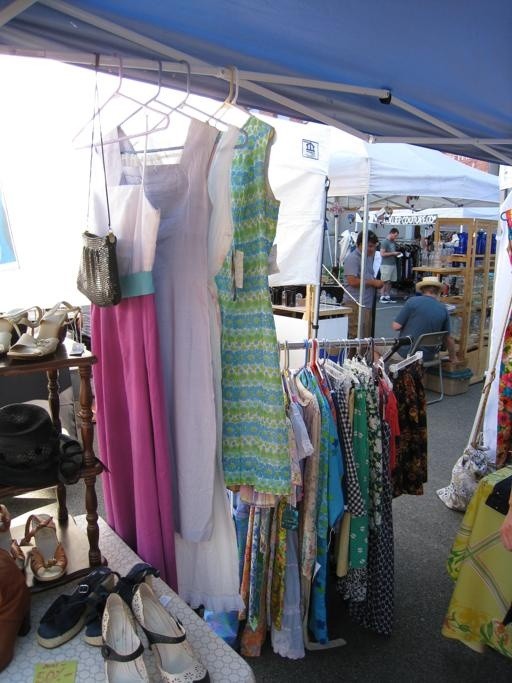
[421,360,471,397]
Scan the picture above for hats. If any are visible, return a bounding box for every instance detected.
[0,402,82,489]
[415,276,444,294]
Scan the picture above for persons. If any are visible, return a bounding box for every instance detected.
[379,228,402,304]
[343,230,384,363]
[498,486,511,555]
[371,275,462,372]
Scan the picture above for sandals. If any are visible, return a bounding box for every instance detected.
[0,305,43,355]
[0,504,25,572]
[6,301,82,361]
[83,562,153,647]
[20,513,68,583]
[35,567,114,650]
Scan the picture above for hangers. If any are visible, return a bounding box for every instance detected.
[279,335,423,418]
[67,51,278,153]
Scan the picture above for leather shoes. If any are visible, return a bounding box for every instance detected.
[131,582,211,683]
[100,592,150,683]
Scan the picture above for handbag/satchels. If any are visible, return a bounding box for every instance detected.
[435,432,494,513]
[75,229,121,308]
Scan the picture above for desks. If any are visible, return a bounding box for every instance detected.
[1,514,255,683]
[274,300,353,319]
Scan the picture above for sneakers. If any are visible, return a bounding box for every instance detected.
[378,297,398,305]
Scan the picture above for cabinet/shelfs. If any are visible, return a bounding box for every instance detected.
[2,334,106,594]
[434,217,498,385]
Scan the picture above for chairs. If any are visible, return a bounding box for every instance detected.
[412,331,448,405]
